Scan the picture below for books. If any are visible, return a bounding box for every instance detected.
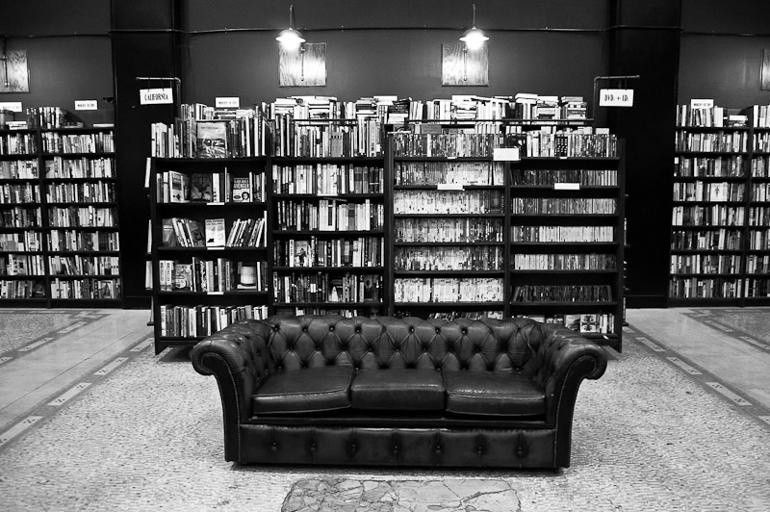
[150,93,620,338]
[0,106,121,302]
[667,104,770,300]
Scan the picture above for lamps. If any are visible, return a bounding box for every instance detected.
[458,4,489,50]
[275,4,306,54]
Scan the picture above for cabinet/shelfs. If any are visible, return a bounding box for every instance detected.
[668,103,770,305]
[1,108,115,308]
[144,94,630,356]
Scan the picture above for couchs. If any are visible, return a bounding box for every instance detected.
[188,316,607,473]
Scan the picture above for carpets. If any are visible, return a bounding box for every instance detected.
[281,478,521,512]
